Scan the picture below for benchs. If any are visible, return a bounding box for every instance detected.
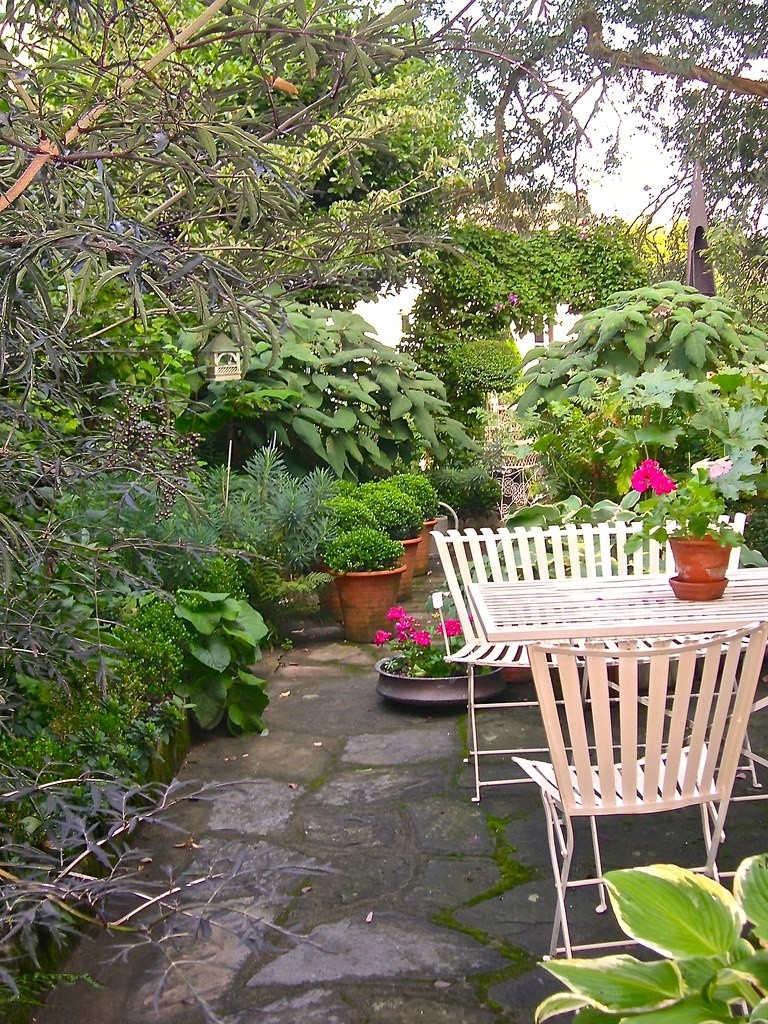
[428,514,761,802]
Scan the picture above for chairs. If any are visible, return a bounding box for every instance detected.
[511,619,768,1014]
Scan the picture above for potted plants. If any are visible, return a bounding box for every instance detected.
[311,474,441,645]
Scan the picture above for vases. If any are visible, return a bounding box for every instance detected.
[430,515,449,556]
[375,653,507,711]
[668,534,732,583]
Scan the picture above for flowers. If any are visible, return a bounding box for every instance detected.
[373,606,492,678]
[623,456,746,556]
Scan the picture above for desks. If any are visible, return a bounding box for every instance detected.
[466,566,768,914]
